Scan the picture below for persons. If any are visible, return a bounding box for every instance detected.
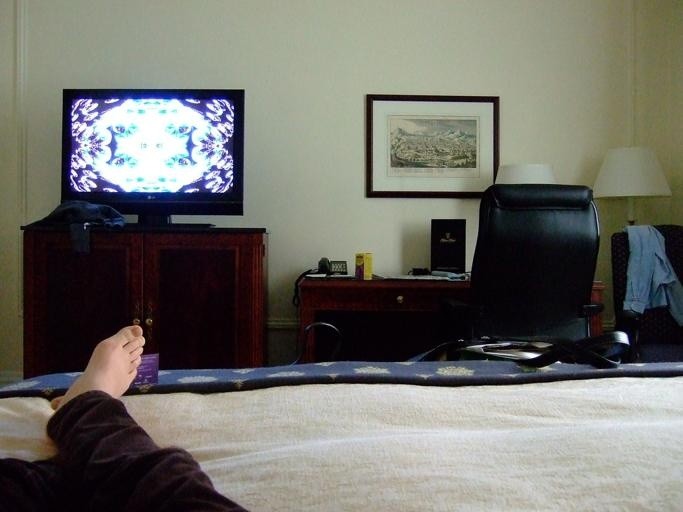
[1,326,252,512]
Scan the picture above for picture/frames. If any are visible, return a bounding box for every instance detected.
[366,94,500,198]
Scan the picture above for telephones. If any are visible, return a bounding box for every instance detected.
[318,257,347,275]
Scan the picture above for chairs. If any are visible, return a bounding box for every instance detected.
[447,184,604,359]
[611,225,682,361]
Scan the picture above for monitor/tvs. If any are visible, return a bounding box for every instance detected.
[62,89,244,232]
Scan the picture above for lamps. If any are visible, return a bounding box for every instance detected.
[593,147,672,225]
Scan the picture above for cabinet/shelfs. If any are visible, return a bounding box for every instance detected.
[297,272,607,363]
[19,225,270,385]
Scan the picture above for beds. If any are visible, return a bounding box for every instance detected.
[0,363,683,512]
